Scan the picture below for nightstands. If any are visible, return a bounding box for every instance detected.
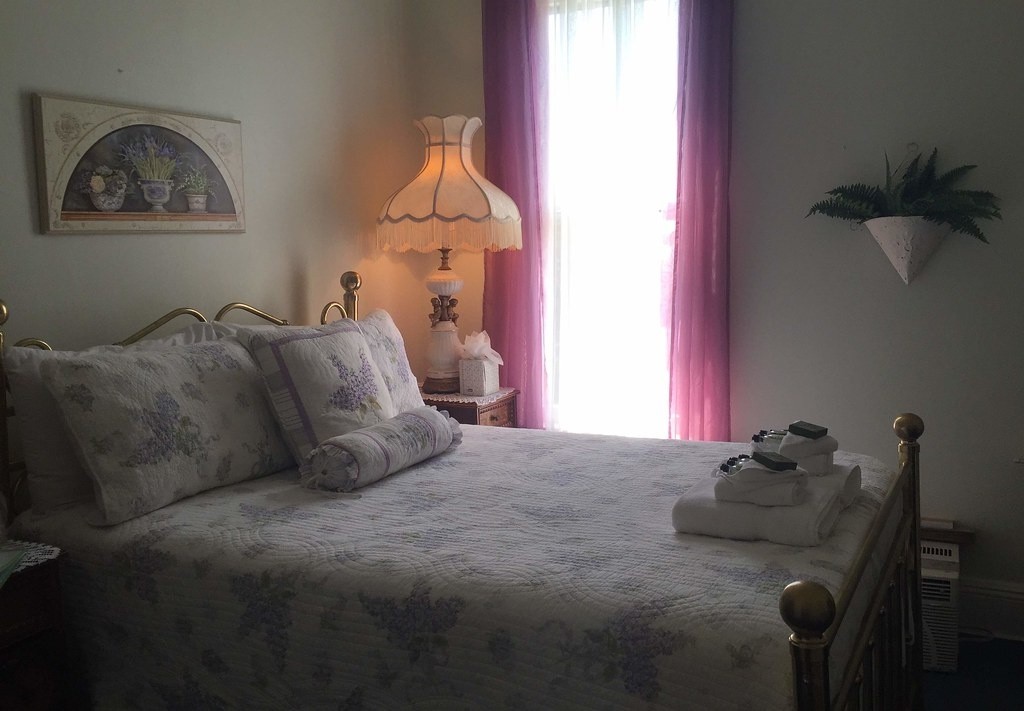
[418,386,522,428]
[0,539,65,711]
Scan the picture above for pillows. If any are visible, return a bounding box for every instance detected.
[247,316,393,465]
[356,308,424,417]
[4,344,88,524]
[298,405,463,490]
[38,334,293,528]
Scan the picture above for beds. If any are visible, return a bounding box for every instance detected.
[0,271,926,711]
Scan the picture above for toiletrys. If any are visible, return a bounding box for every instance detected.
[752,421,828,443]
[720,451,798,475]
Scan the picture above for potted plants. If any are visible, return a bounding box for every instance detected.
[803,146,1002,285]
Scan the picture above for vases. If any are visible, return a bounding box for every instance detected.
[138,179,174,213]
[90,193,125,213]
[185,194,209,214]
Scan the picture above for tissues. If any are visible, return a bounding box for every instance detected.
[454,330,504,396]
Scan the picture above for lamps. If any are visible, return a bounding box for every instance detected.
[373,113,523,393]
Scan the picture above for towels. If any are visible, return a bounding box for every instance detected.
[671,434,862,548]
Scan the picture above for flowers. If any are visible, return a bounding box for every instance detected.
[118,133,190,178]
[79,165,135,195]
[177,163,218,203]
